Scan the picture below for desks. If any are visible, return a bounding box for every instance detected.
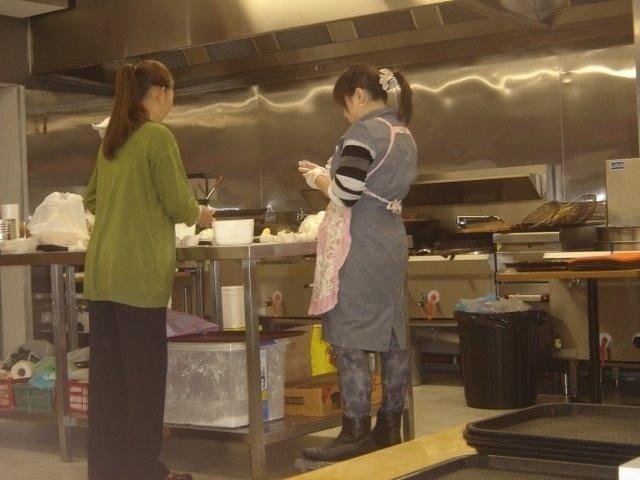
[282,415,484,480]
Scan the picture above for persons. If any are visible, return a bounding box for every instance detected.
[296,60,419,462]
[81,58,214,480]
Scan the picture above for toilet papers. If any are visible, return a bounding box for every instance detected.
[12,360,34,380]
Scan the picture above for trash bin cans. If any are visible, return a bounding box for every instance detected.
[453,309,549,410]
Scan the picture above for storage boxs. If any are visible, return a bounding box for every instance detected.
[284,372,384,417]
[163,333,290,429]
[284,325,338,383]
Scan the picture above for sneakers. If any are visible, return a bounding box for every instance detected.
[167,472,193,480]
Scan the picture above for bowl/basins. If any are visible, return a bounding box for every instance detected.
[211,218,255,246]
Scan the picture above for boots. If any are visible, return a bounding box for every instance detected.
[303,414,377,462]
[372,410,403,449]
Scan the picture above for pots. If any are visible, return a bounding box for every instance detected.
[398,214,441,240]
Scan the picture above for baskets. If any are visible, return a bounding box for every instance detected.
[65,379,90,421]
[0,377,55,416]
